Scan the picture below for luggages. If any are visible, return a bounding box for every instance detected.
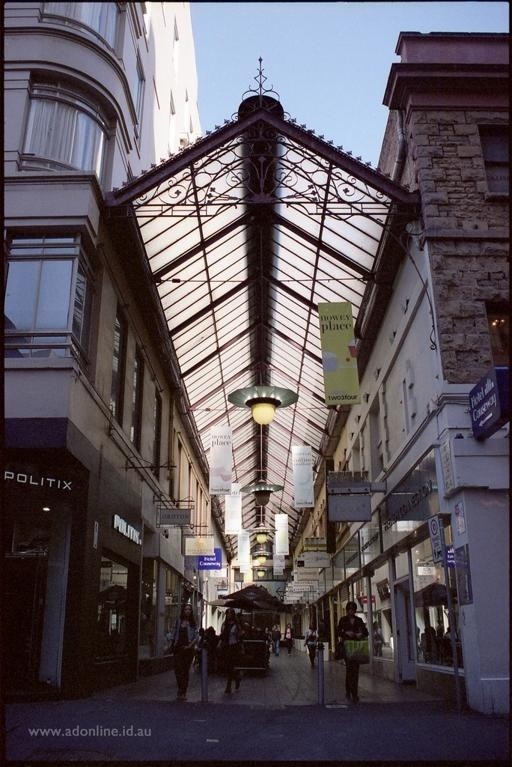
[241,650,266,677]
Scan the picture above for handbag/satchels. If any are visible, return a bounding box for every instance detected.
[334,641,344,660]
[345,640,371,664]
[212,646,235,662]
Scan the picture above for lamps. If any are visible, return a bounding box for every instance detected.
[238,426,285,578]
[228,359,299,426]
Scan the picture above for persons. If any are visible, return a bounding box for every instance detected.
[270,623,282,655]
[337,599,369,706]
[373,620,385,656]
[192,623,215,669]
[216,606,247,698]
[415,619,459,664]
[283,621,294,656]
[303,622,319,667]
[170,601,199,700]
